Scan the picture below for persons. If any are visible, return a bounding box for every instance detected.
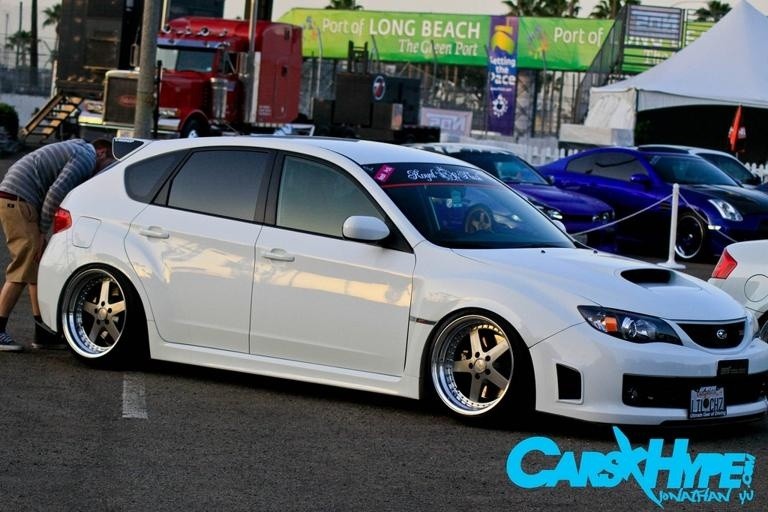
[0,138,114,351]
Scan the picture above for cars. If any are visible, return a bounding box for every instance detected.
[34,133,768,430]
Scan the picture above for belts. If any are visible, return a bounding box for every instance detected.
[0,190,27,203]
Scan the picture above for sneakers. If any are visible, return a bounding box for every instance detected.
[0,331,23,351]
[32,333,68,350]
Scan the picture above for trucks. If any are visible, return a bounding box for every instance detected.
[131,15,442,142]
